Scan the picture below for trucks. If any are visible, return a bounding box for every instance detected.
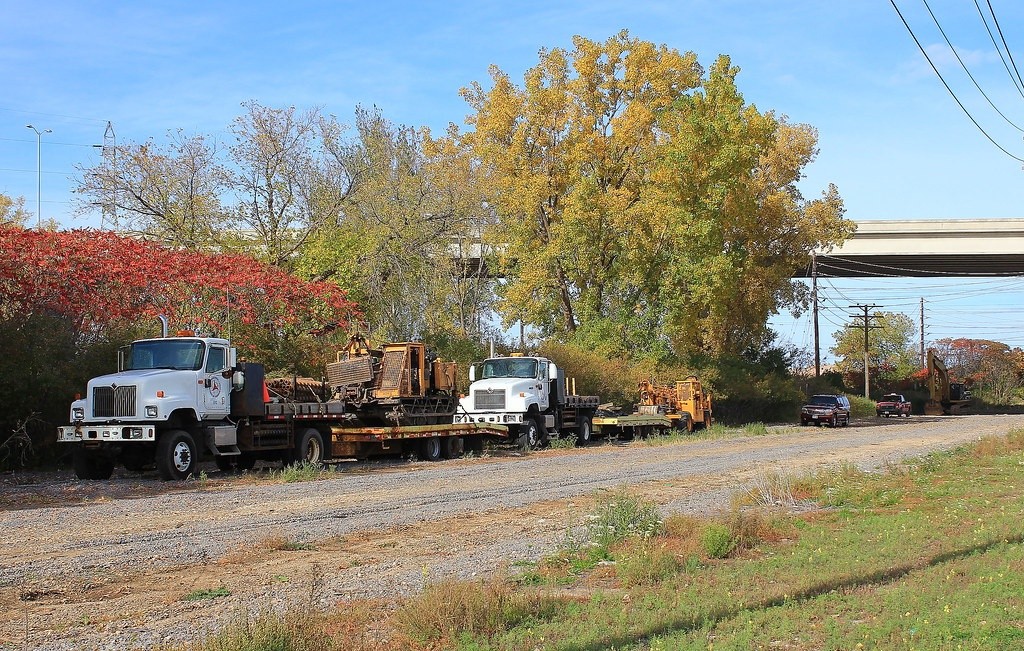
[56,313,346,482]
[592,376,714,440]
[455,337,600,453]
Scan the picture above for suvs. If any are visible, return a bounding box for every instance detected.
[801,394,851,427]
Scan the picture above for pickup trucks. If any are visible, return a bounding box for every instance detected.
[876,393,912,418]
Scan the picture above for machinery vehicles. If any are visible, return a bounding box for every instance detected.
[264,321,461,426]
